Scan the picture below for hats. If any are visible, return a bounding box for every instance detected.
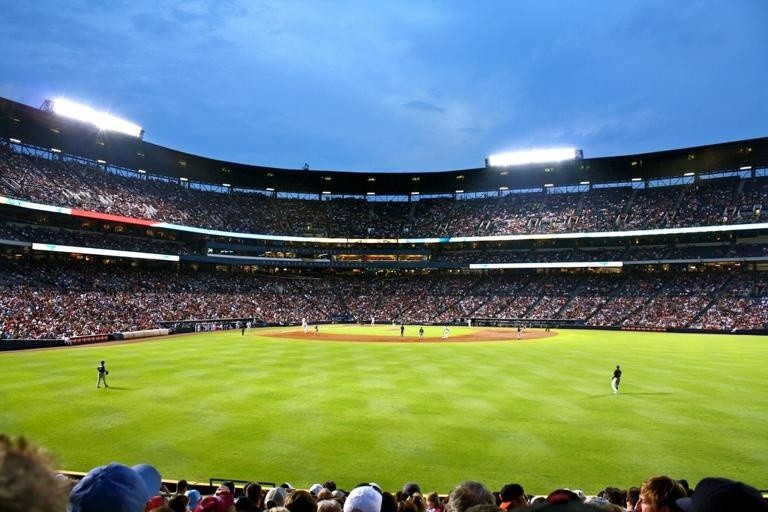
[342,481,384,512]
[675,476,763,512]
[185,489,202,510]
[264,482,323,509]
[67,462,162,512]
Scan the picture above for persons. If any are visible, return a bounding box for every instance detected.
[95,359,108,387]
[611,365,622,394]
[1,144,768,343]
[1,428,768,512]
